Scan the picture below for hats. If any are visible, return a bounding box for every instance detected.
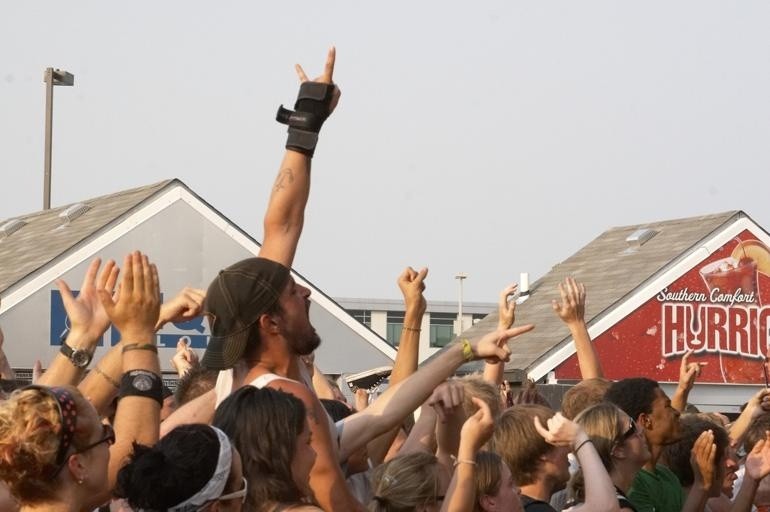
[198,257,291,370]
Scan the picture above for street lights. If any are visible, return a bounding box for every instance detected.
[42,65,74,209]
[455,274,466,335]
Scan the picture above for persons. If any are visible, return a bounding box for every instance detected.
[0,44,770,512]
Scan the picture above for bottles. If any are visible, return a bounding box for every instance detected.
[699,255,769,384]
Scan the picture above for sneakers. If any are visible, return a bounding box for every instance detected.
[344,365,393,396]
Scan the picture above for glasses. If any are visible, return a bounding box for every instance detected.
[196,475,248,512]
[609,417,639,458]
[661,430,700,464]
[56,424,115,479]
